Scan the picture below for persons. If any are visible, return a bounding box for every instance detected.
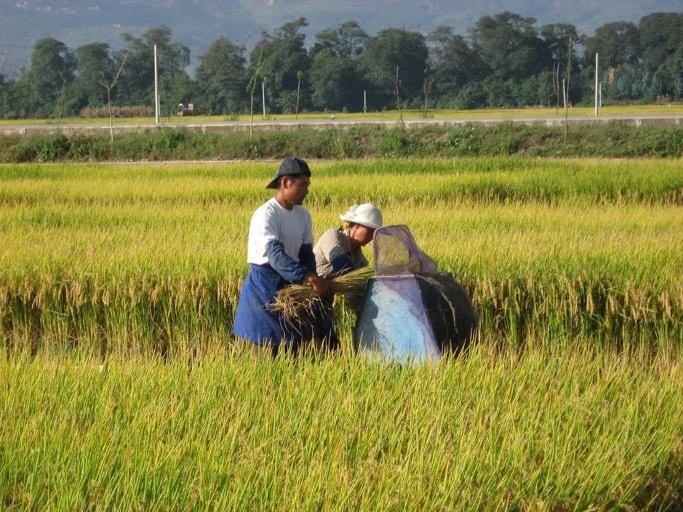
[231,157,330,356]
[312,202,383,350]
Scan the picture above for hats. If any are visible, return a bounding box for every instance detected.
[265,158,311,188]
[340,203,383,229]
